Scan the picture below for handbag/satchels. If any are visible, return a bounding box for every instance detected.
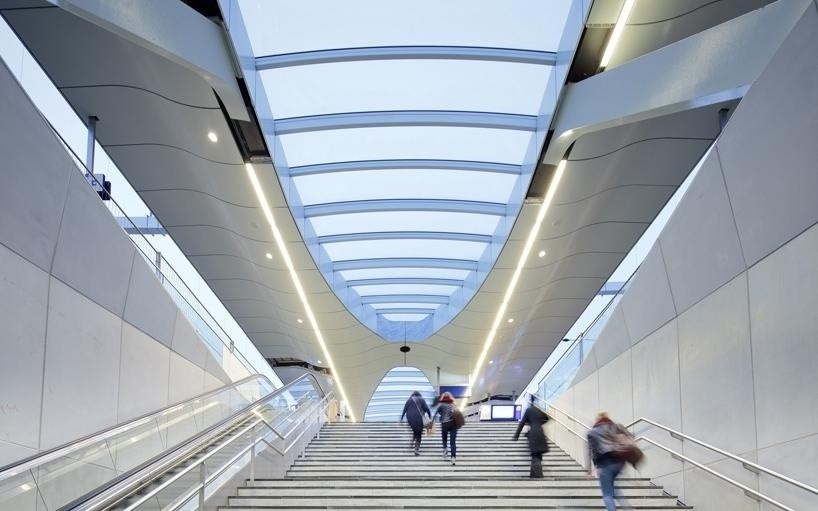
[610,432,643,468]
[421,412,431,427]
[451,408,465,429]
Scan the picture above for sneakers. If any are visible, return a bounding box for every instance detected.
[442,448,448,458]
[450,456,456,467]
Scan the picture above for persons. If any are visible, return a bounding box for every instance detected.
[584,411,645,511]
[513,394,551,478]
[431,391,458,465]
[400,391,430,455]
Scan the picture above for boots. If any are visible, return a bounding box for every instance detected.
[413,442,420,457]
